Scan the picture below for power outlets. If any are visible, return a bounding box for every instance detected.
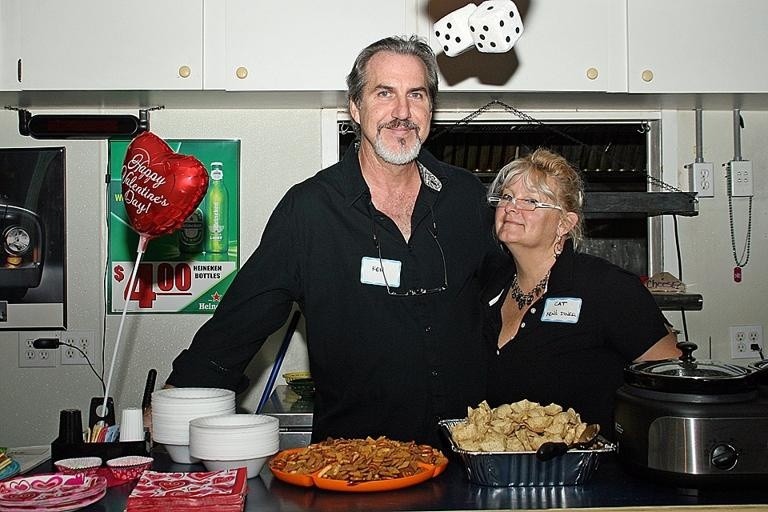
[18,331,60,368]
[729,325,764,359]
[60,329,96,365]
[728,161,754,200]
[688,162,715,198]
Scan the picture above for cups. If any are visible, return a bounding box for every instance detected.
[577,238,646,276]
[117,405,145,443]
[57,408,84,443]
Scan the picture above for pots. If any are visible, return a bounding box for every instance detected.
[608,336,768,469]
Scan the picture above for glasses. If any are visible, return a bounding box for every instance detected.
[486,193,562,211]
[378,227,447,297]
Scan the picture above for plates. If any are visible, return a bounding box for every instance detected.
[144,385,282,478]
[0,475,108,512]
[264,443,449,493]
[0,459,21,481]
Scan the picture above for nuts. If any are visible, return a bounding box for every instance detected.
[269,435,445,484]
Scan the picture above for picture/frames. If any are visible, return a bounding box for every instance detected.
[104,138,244,317]
[0,145,71,333]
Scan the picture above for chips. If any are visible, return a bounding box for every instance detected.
[449,397,585,452]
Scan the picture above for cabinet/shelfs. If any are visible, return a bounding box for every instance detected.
[0,1,413,96]
[415,1,767,94]
[580,190,702,311]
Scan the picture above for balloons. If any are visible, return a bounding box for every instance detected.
[120,129,213,240]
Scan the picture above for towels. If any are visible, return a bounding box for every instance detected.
[127,467,248,512]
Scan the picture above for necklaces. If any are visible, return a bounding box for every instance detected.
[725,161,752,283]
[505,265,555,312]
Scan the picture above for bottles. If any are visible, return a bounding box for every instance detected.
[204,161,229,253]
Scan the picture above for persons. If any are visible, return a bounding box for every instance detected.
[473,146,684,433]
[140,33,513,445]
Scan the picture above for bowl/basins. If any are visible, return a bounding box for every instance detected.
[105,456,153,481]
[54,457,102,476]
